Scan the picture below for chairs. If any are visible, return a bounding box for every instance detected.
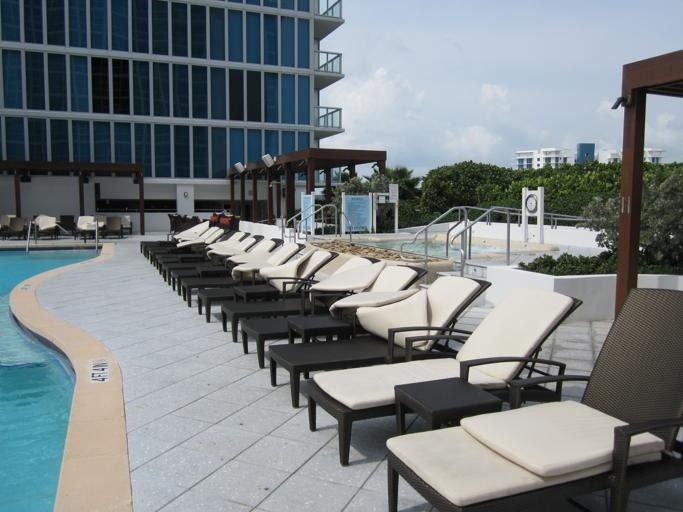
[0,215,132,244]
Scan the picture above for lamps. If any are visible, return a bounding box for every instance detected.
[233,162,246,174]
[261,153,276,168]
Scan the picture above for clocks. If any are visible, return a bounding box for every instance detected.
[525,194,537,213]
